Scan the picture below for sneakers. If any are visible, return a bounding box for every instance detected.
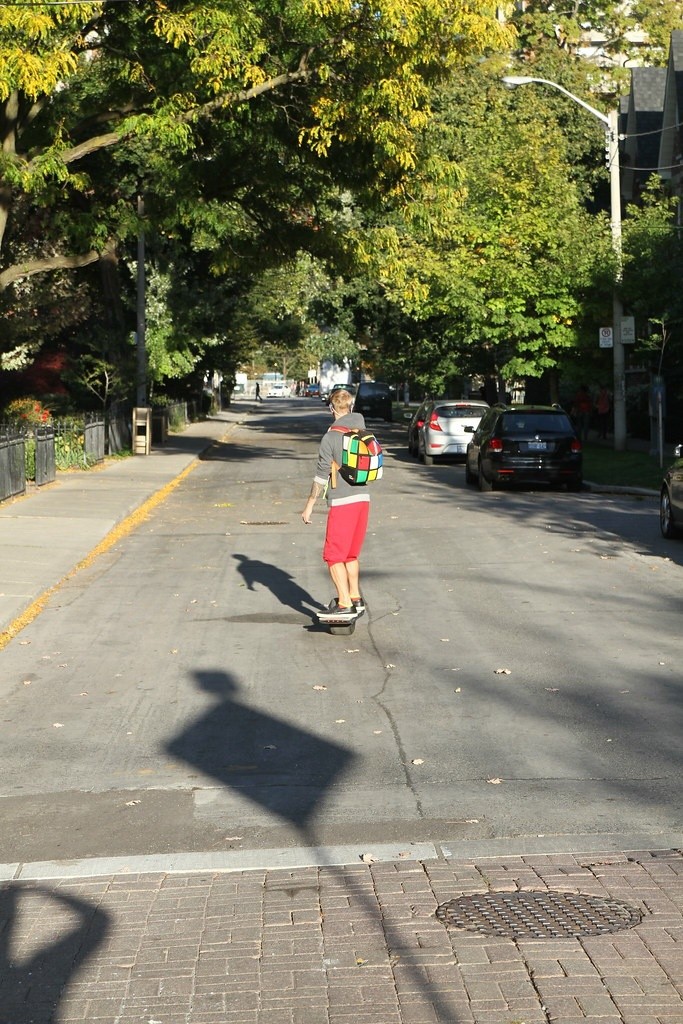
[324,597,366,611]
[316,604,358,618]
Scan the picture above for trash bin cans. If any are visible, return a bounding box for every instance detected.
[131,407,153,456]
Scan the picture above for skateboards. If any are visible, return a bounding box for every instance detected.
[318,604,365,635]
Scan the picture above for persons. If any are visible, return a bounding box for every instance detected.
[301,390,369,617]
[256,382,262,400]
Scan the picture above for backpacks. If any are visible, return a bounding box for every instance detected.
[327,427,383,486]
